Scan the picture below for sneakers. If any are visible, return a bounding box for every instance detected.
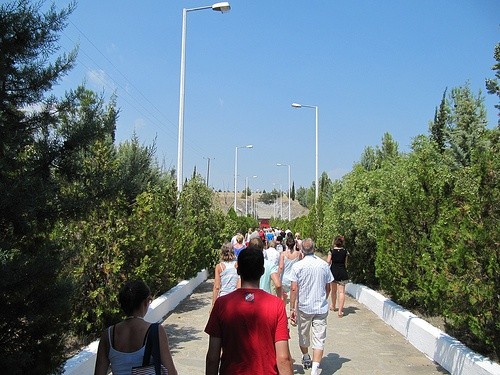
[302,355,312,369]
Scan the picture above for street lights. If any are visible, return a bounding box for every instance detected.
[277,163,291,224]
[249,183,279,222]
[292,102,320,232]
[176,0,231,212]
[272,183,283,222]
[234,144,253,214]
[245,176,257,217]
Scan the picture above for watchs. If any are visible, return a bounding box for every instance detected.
[290,308,295,311]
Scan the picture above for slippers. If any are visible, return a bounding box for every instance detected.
[338,312,344,317]
[329,306,336,311]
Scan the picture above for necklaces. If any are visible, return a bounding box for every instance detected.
[124,316,145,320]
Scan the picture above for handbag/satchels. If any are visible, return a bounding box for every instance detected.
[131,323,169,375]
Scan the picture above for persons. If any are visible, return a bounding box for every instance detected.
[211,243,237,307]
[231,231,246,247]
[245,226,303,253]
[94,278,178,375]
[265,239,280,266]
[204,247,294,375]
[237,237,282,299]
[328,236,351,317]
[288,237,335,375]
[232,236,247,257]
[277,238,302,308]
